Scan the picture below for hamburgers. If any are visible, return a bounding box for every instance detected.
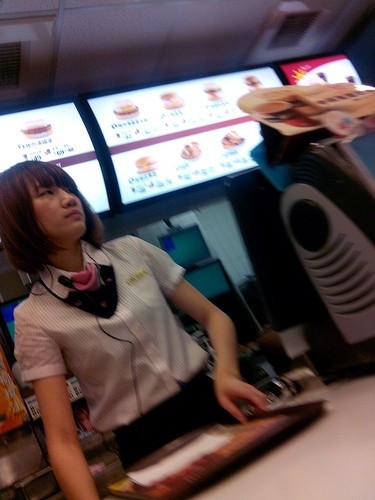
[135,156,157,173]
[203,82,225,101]
[244,75,263,92]
[113,100,139,120]
[161,92,183,110]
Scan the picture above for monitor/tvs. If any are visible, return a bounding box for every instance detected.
[1,295,30,357]
[158,225,211,270]
[105,400,327,500]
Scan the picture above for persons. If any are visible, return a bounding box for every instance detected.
[0,159,269,500]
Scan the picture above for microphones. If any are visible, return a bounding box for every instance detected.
[58,275,103,306]
